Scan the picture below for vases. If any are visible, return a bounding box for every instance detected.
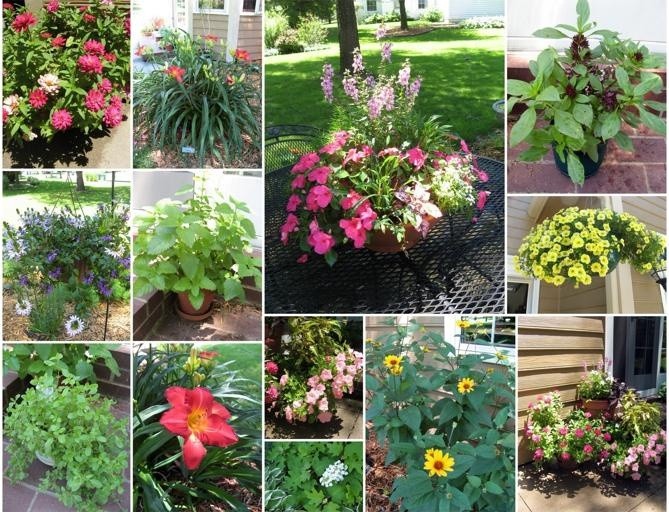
[556,459,579,470]
[60,259,91,283]
[581,397,608,416]
[549,119,608,181]
[574,237,623,277]
[364,202,444,253]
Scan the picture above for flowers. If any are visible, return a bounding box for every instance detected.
[507,0,666,189]
[577,357,613,400]
[524,387,611,473]
[611,429,667,480]
[2,198,131,298]
[513,207,667,289]
[281,22,492,267]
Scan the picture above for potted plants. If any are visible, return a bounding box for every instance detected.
[133,171,262,320]
[2,353,130,512]
